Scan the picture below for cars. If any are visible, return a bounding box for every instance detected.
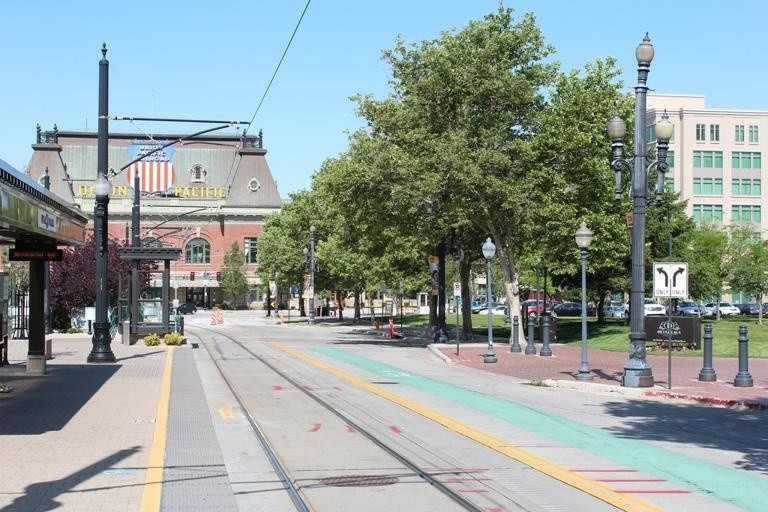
[170,302,197,315]
[328,301,338,311]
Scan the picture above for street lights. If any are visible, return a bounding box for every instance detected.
[575,221,593,373]
[87,41,118,364]
[482,237,496,363]
[303,226,320,322]
[608,31,673,388]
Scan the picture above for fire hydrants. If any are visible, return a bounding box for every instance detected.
[374,319,382,330]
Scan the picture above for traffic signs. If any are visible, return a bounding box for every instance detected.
[652,261,688,299]
